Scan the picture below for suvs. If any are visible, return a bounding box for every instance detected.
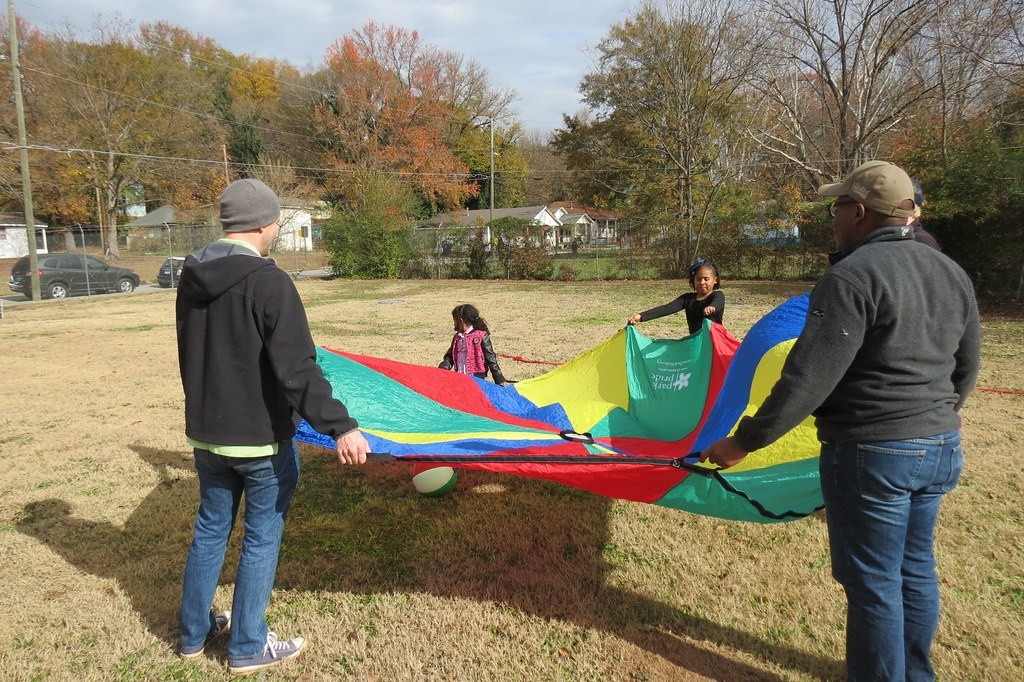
[9,252,141,300]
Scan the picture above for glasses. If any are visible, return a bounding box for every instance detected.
[829,199,870,217]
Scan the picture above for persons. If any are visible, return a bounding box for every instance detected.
[175,178,372,674]
[437,304,508,387]
[699,160,980,682]
[572,238,577,259]
[906,177,941,253]
[629,258,725,335]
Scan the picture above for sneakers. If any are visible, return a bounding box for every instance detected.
[181,608,233,658]
[229,633,306,676]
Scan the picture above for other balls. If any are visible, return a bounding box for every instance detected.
[412,462,458,497]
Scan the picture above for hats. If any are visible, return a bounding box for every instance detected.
[817,160,915,218]
[910,177,923,208]
[219,180,280,234]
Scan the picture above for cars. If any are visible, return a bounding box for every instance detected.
[157,257,186,288]
